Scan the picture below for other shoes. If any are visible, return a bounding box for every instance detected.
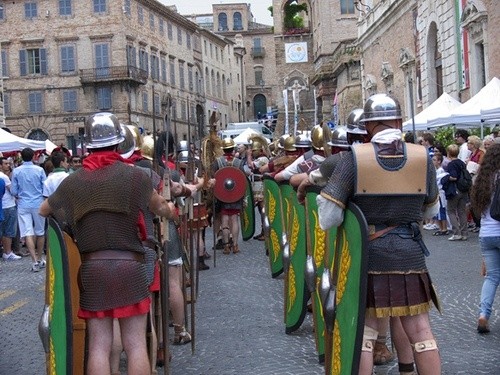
[222,247,230,254]
[253,232,266,240]
[372,341,394,365]
[156,347,172,367]
[173,332,192,344]
[15,251,29,257]
[233,245,240,253]
[186,295,192,303]
[212,240,224,249]
[307,304,313,313]
[423,221,479,241]
[199,263,210,271]
[229,236,233,245]
[204,253,211,259]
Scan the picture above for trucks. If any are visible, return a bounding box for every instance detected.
[227,122,273,142]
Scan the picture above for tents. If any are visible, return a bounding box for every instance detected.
[0,128,46,152]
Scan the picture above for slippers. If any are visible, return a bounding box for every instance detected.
[476,324,490,334]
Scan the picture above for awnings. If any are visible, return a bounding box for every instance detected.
[402,77,500,139]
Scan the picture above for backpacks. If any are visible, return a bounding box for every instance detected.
[452,161,472,193]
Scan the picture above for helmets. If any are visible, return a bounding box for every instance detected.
[251,140,263,150]
[176,140,190,163]
[277,93,408,151]
[82,111,154,160]
[220,137,236,149]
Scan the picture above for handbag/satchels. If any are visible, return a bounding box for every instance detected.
[490,179,500,221]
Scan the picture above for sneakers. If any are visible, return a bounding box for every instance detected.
[32,263,39,272]
[38,260,46,268]
[2,252,22,260]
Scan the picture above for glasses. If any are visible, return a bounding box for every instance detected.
[72,160,81,163]
[455,136,461,139]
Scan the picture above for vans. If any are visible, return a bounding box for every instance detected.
[220,129,271,144]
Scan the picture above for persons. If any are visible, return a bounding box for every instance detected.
[0,92,500,375]
